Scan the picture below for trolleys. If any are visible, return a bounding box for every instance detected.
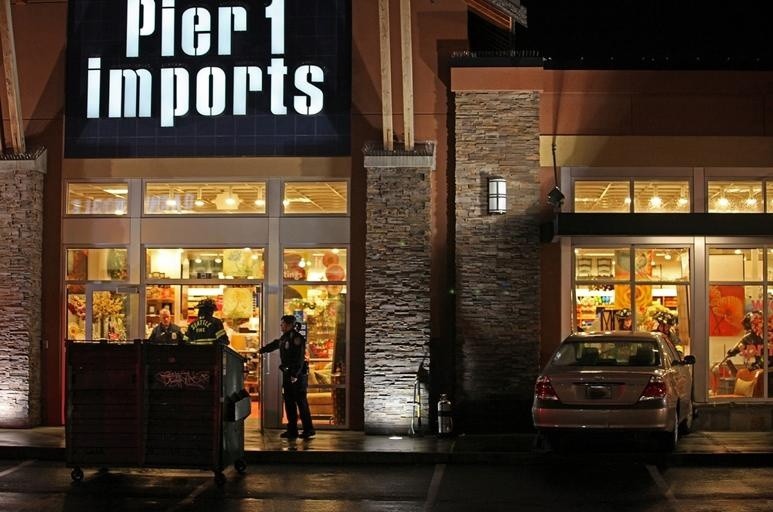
[60,337,252,488]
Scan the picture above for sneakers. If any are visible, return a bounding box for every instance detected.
[280,431,315,438]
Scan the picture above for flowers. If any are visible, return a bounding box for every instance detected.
[640,302,677,325]
[738,312,773,361]
[68,291,128,341]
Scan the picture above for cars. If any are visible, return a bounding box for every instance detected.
[529,324,698,449]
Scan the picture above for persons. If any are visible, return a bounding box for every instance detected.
[149,309,184,344]
[251,315,316,437]
[727,314,773,397]
[183,299,230,345]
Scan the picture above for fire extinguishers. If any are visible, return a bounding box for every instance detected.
[438,393,454,434]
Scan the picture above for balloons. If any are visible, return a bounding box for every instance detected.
[322,252,346,297]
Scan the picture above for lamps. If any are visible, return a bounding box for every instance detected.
[547,186,565,208]
[488,178,507,215]
